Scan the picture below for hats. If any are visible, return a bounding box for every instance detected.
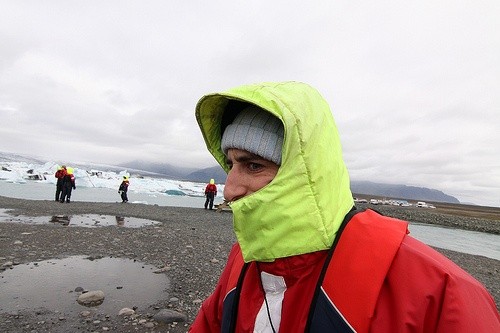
[220,109,284,167]
[209,179,214,185]
[67,167,73,174]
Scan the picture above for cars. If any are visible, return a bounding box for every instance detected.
[353,197,437,209]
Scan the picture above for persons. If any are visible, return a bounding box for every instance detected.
[55,165,76,203]
[204,179,217,210]
[194,79,500,333]
[118,176,129,203]
